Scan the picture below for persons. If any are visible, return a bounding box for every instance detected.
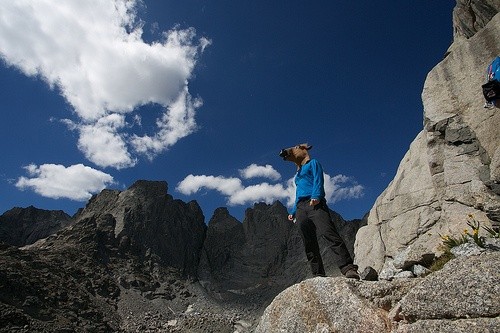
[280,143,360,278]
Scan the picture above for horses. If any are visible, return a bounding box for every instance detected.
[280,143,312,167]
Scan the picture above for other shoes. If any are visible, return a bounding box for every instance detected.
[346,270,360,280]
